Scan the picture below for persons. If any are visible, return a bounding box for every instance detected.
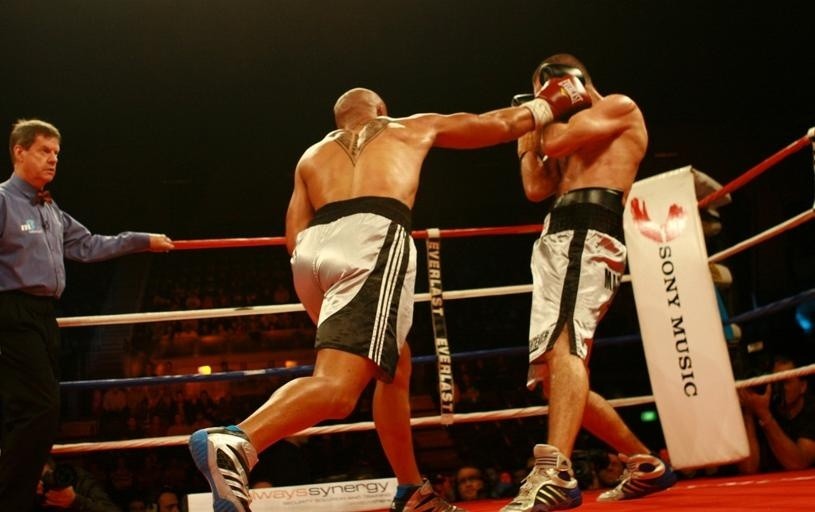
[736,356,815,476]
[0,119,174,512]
[189,72,591,512]
[38,271,316,510]
[433,297,624,501]
[499,55,680,512]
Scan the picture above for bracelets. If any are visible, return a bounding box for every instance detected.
[757,412,772,427]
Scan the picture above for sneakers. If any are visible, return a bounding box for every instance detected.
[497,444,583,512]
[596,453,678,503]
[188,423,260,512]
[389,477,467,512]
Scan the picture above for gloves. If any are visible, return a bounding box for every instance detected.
[509,64,593,159]
